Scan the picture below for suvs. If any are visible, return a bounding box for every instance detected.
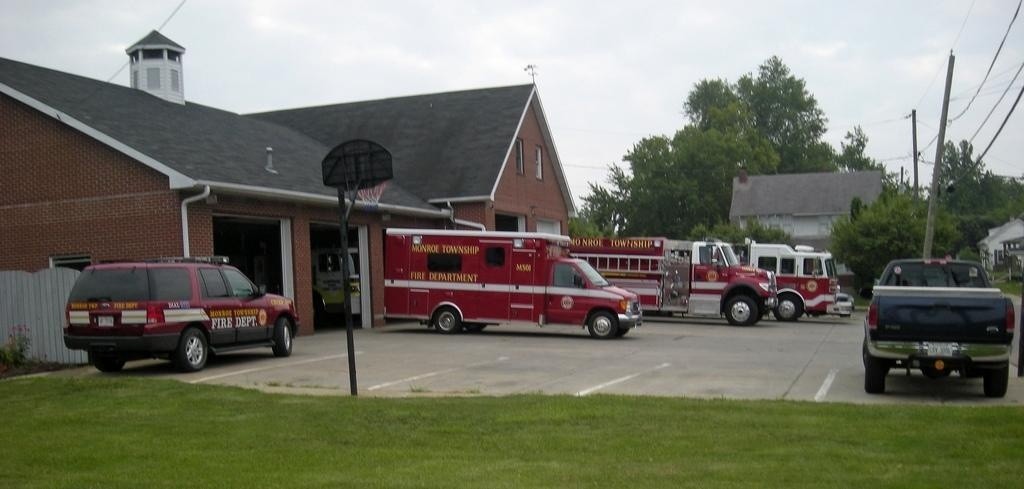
[62,255,300,371]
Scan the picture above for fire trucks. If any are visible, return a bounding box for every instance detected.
[381,226,643,340]
[566,234,779,326]
[725,237,840,322]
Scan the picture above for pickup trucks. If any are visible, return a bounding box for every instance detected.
[862,260,1016,399]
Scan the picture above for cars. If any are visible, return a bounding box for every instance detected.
[838,293,855,317]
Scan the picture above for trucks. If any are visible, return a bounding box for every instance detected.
[309,245,359,322]
